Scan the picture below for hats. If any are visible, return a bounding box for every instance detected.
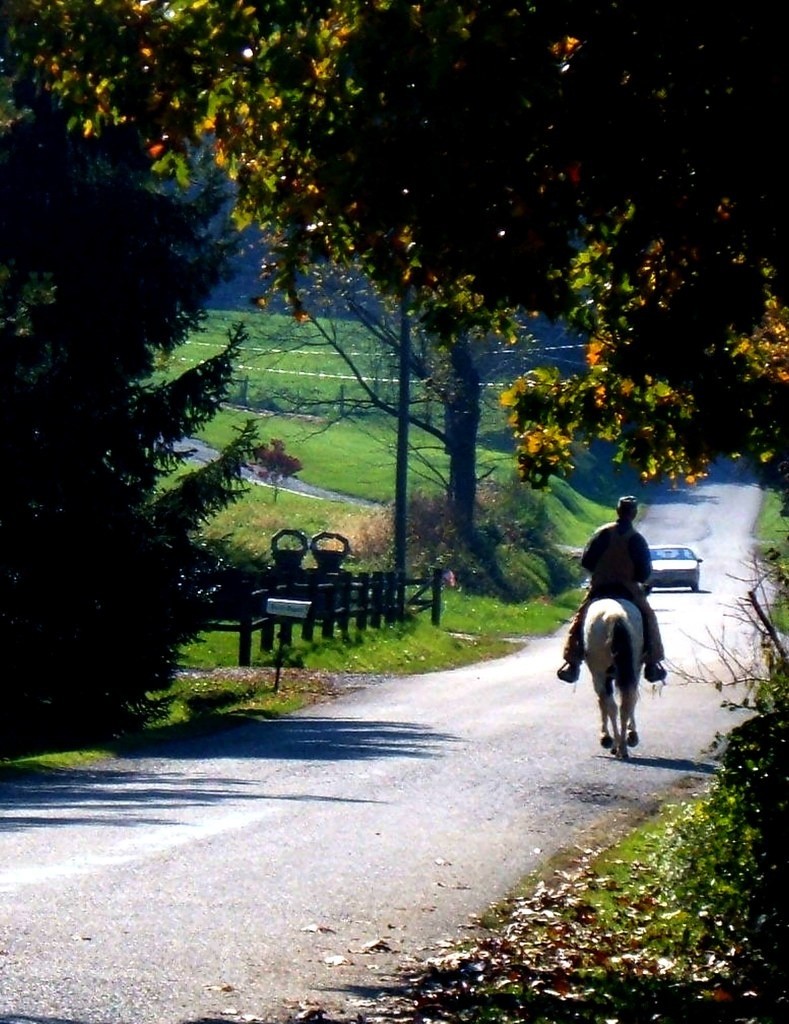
[617,494,638,510]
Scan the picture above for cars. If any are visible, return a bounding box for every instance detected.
[642,545,703,596]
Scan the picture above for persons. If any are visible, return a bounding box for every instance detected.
[558,495,667,684]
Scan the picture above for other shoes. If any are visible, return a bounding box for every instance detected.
[559,663,580,683]
[644,665,667,682]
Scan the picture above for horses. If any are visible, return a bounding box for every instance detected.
[581,597,645,761]
